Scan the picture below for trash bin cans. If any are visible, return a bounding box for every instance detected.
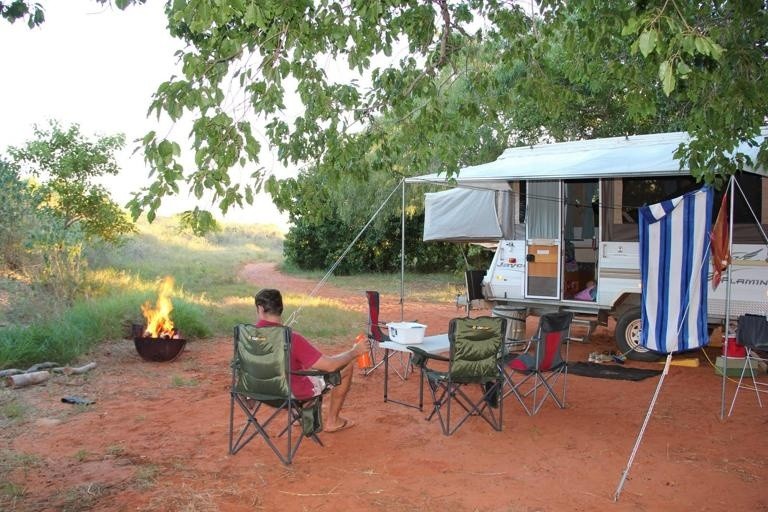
[466,268,489,300]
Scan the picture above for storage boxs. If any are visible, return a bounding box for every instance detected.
[713,334,759,378]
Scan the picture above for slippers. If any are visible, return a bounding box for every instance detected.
[323,418,355,433]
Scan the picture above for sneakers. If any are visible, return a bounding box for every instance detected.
[588,350,613,363]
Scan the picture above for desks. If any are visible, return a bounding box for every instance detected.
[378,333,452,413]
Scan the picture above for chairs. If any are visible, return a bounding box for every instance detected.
[362,291,415,380]
[406,311,576,436]
[227,323,347,466]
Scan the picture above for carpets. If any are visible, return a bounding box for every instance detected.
[551,359,663,382]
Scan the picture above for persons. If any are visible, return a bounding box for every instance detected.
[255,288,372,432]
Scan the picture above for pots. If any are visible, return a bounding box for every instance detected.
[134,336,186,364]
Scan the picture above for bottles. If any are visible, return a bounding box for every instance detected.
[355,332,373,371]
